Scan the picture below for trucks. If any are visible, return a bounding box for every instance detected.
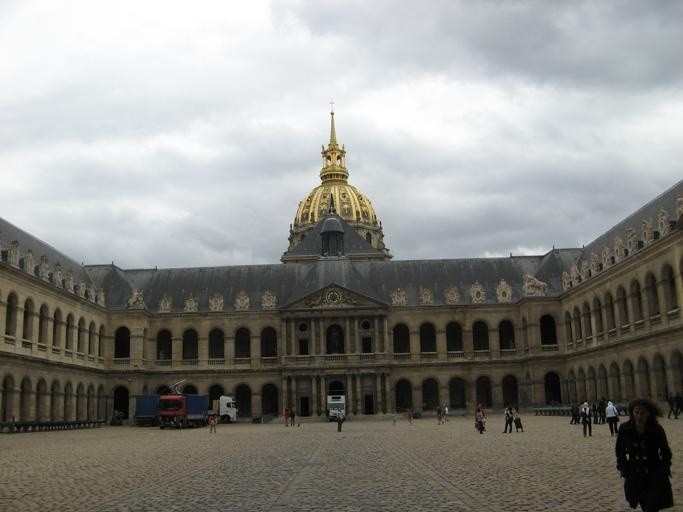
[157,391,209,428]
[133,393,238,428]
[325,394,346,421]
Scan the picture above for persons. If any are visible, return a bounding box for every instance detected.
[436,405,449,425]
[209,414,217,433]
[336,407,345,432]
[391,411,397,426]
[502,403,513,433]
[407,408,413,424]
[285,407,295,427]
[475,401,487,434]
[570,391,683,437]
[513,404,524,432]
[615,397,674,512]
